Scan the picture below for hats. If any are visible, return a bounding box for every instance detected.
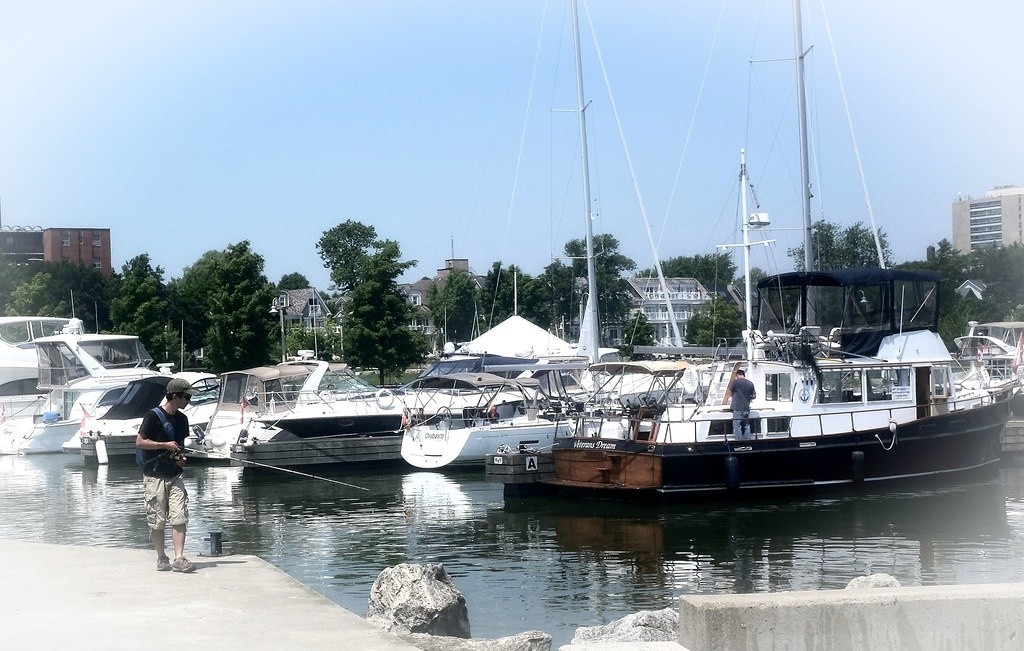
[164,378,200,396]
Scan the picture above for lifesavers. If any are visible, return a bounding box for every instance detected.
[375,388,393,409]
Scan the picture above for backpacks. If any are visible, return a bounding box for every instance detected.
[135,408,187,474]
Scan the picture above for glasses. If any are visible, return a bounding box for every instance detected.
[180,394,194,402]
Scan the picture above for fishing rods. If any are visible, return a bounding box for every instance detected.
[180,445,369,492]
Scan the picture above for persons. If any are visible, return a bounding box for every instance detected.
[502,400,509,405]
[136,378,198,572]
[485,405,499,424]
[729,370,756,440]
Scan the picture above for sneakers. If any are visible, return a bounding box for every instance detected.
[157,558,173,571]
[173,558,198,573]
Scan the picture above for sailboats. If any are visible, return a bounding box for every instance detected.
[485,146,1020,513]
[401,0,705,471]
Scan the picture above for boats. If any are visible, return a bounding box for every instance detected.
[0,290,161,458]
[80,361,258,459]
[947,320,1023,419]
[184,349,380,459]
[0,316,84,422]
[252,271,685,440]
